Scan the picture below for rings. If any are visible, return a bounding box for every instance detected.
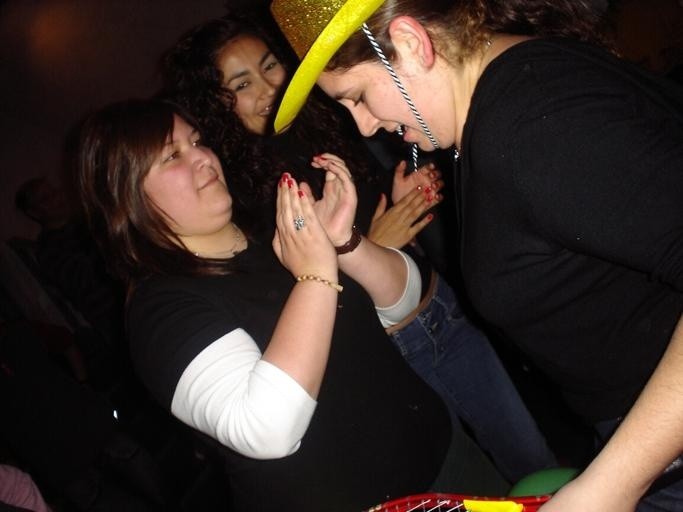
[292,215,305,232]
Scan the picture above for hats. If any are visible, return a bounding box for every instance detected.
[271,0,384,138]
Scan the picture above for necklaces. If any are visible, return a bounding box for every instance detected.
[189,220,242,258]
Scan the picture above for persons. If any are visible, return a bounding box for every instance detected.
[0,318,170,512]
[264,1,681,512]
[74,96,515,511]
[13,173,158,424]
[151,14,561,484]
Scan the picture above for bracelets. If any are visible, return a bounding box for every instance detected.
[332,221,363,256]
[294,273,345,294]
